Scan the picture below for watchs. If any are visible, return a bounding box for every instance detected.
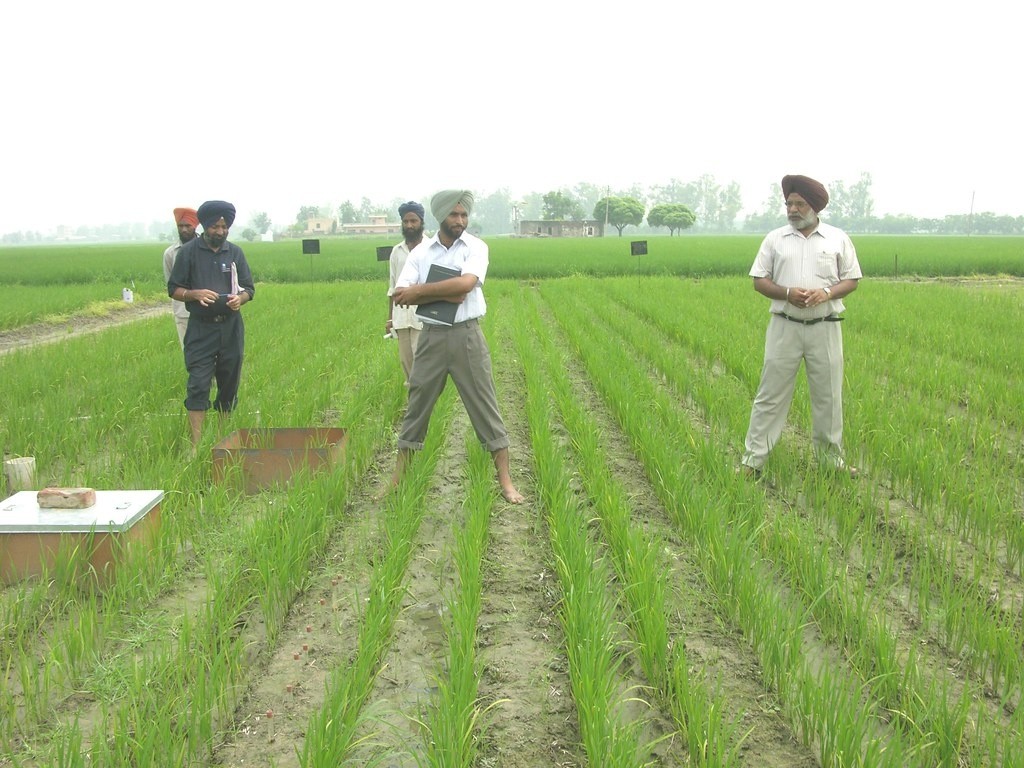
[823,286,832,299]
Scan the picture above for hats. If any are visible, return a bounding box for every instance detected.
[174,208,199,229]
[431,190,474,223]
[398,201,424,220]
[197,201,236,229]
[783,175,829,213]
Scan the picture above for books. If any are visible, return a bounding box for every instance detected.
[414,262,463,327]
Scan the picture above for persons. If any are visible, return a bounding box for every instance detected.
[384,200,430,387]
[381,188,527,504]
[162,207,202,353]
[736,174,863,481]
[167,200,256,456]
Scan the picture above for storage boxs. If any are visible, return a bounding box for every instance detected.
[208,427,349,496]
[0,489,165,598]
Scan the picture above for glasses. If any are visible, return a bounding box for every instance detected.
[786,200,809,208]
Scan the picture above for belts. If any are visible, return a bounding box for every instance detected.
[189,313,231,323]
[777,313,845,325]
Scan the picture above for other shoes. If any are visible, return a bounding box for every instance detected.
[736,465,759,478]
[840,463,857,477]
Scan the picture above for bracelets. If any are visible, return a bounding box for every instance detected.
[182,289,189,297]
[386,319,393,322]
[786,287,790,302]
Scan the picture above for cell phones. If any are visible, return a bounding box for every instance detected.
[216,293,228,303]
